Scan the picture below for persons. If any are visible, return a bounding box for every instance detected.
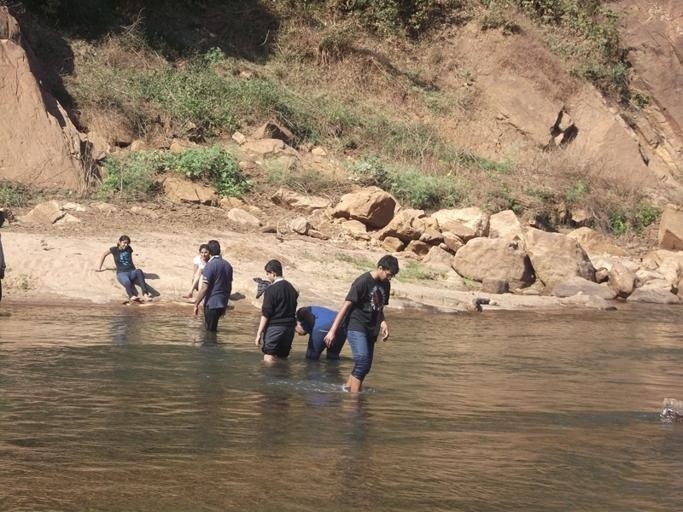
[323,255,399,392]
[0,211,12,316]
[96,235,151,302]
[294,305,347,361]
[254,259,299,365]
[182,244,210,299]
[193,240,233,332]
